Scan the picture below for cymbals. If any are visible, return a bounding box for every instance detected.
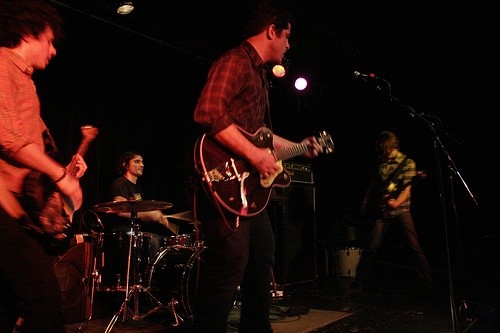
[92,199,174,214]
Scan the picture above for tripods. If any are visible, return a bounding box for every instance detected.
[105,204,188,333]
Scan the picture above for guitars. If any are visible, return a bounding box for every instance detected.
[193,126,336,218]
[362,170,427,221]
[20,124,99,243]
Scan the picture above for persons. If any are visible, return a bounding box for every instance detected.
[0,0,87,333]
[109,151,144,201]
[359,131,432,273]
[192,0,323,333]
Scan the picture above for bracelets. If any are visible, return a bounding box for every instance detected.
[55,169,67,183]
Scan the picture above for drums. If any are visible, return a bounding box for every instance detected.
[323,244,364,284]
[47,244,100,324]
[142,245,216,321]
[161,233,195,247]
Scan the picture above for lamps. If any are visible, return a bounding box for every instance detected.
[113,0,137,16]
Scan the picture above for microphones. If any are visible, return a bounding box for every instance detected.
[94,210,104,230]
[354,71,386,82]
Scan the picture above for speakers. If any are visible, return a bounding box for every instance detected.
[47,238,92,324]
[266,184,317,286]
